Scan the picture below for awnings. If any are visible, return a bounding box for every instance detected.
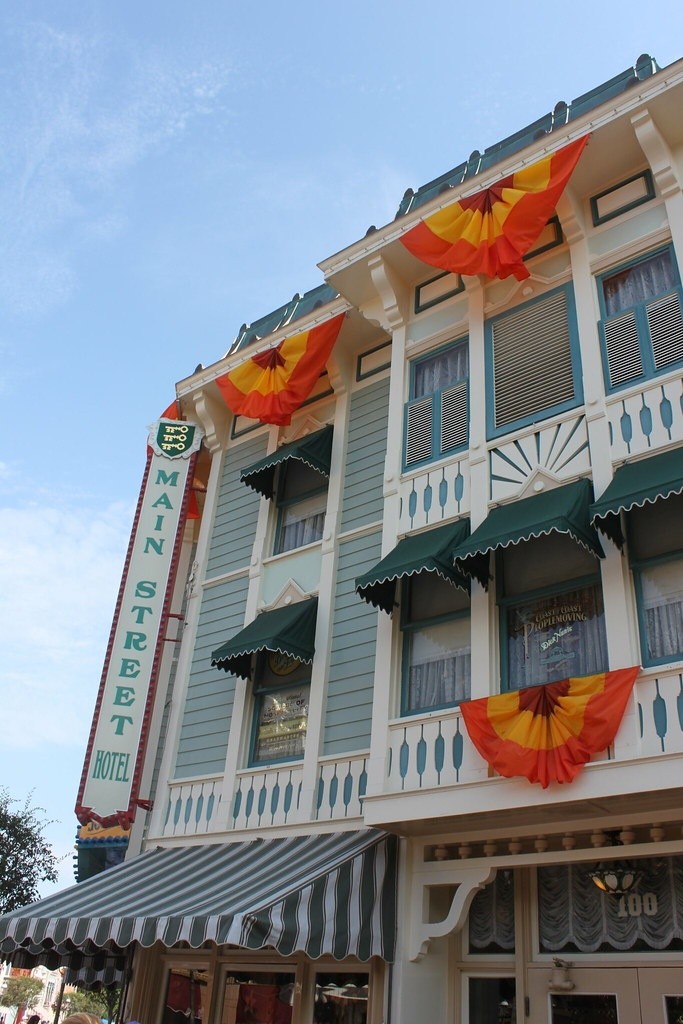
[452,478,607,593]
[63,968,128,993]
[0,828,401,973]
[590,446,683,556]
[240,424,334,503]
[355,516,471,616]
[210,597,318,682]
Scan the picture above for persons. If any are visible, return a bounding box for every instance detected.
[0,1016,5,1024]
[60,1012,104,1024]
[27,1014,50,1024]
